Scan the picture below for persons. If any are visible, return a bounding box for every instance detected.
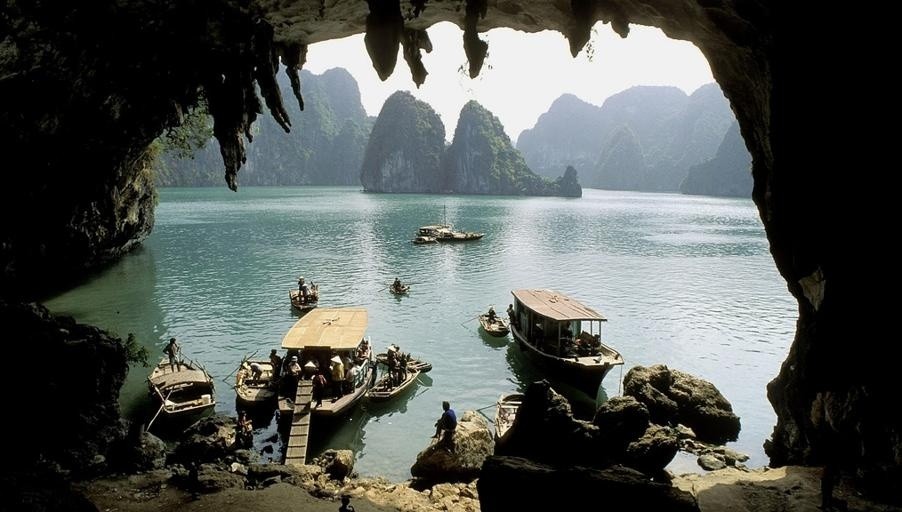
[246,360,263,385]
[384,342,408,389]
[506,304,514,319]
[487,305,497,324]
[562,320,601,357]
[270,338,372,408]
[393,277,410,294]
[163,337,181,372]
[339,495,354,512]
[431,401,457,438]
[238,411,252,433]
[297,275,319,304]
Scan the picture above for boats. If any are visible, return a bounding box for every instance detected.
[508,284,624,402]
[413,205,486,245]
[479,312,510,340]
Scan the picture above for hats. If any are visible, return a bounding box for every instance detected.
[291,355,299,362]
[304,360,316,368]
[330,355,343,364]
